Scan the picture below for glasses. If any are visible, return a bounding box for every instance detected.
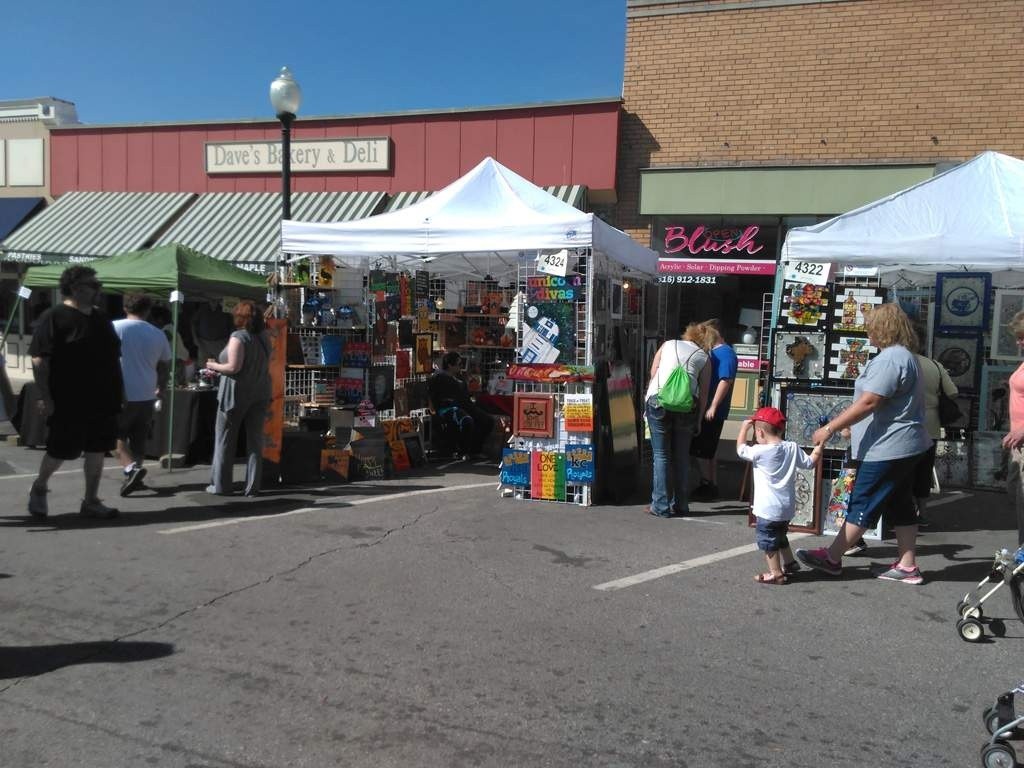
[73,280,102,291]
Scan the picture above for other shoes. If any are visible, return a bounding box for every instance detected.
[29,480,50,516]
[473,458,491,466]
[916,514,929,527]
[436,458,463,470]
[644,506,654,515]
[79,499,119,519]
[247,489,260,498]
[205,484,216,494]
[694,486,713,503]
[708,485,721,498]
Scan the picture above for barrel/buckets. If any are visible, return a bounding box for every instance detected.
[321,336,344,365]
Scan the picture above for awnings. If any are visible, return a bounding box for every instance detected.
[0,197,44,241]
[382,184,589,213]
[150,192,387,276]
[0,191,195,264]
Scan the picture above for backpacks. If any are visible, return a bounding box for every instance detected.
[656,340,704,413]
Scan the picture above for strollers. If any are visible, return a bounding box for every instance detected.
[957,543,1024,643]
[979,684,1024,768]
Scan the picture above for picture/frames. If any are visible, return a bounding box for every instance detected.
[747,450,824,536]
[989,288,1024,361]
[978,363,1016,433]
[415,333,433,374]
[931,332,985,399]
[933,271,992,333]
[512,392,555,440]
[778,386,854,457]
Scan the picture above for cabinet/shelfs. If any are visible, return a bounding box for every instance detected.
[456,312,516,349]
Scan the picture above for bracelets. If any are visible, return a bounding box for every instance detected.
[813,450,821,456]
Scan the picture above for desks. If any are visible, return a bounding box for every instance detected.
[144,386,253,461]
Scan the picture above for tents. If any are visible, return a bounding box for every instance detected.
[280,157,659,507]
[0,242,268,474]
[780,151,1024,274]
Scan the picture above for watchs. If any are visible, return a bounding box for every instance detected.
[824,425,832,435]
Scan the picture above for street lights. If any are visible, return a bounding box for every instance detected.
[269,65,302,272]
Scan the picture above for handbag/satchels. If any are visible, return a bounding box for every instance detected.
[931,358,963,426]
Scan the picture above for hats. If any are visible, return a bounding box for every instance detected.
[751,407,786,432]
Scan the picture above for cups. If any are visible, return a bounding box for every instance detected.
[208,359,214,373]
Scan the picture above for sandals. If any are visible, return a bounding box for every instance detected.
[781,559,801,573]
[755,573,787,584]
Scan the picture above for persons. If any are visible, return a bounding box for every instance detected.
[112,289,171,496]
[795,302,934,585]
[645,318,739,518]
[737,407,825,584]
[910,319,959,525]
[206,301,268,497]
[191,301,232,361]
[253,307,273,360]
[26,265,127,517]
[429,351,494,460]
[1002,309,1024,547]
[843,537,867,556]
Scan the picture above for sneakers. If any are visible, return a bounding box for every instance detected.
[871,563,924,584]
[797,547,843,576]
[120,463,147,497]
